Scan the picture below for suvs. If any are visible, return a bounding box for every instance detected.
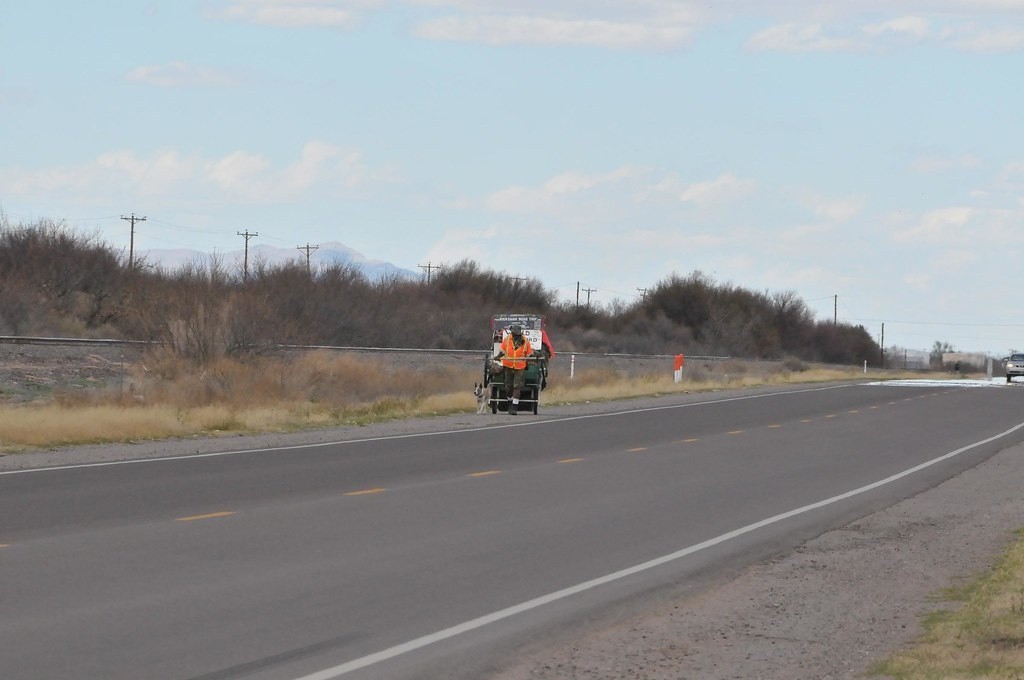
[1002,353,1024,383]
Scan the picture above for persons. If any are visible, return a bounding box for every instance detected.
[493,326,540,416]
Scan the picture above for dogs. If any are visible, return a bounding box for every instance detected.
[474,382,490,415]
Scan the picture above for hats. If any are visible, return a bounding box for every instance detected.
[512,323,522,335]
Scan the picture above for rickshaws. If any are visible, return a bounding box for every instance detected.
[482,313,547,415]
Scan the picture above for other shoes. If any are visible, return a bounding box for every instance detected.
[510,403,519,415]
